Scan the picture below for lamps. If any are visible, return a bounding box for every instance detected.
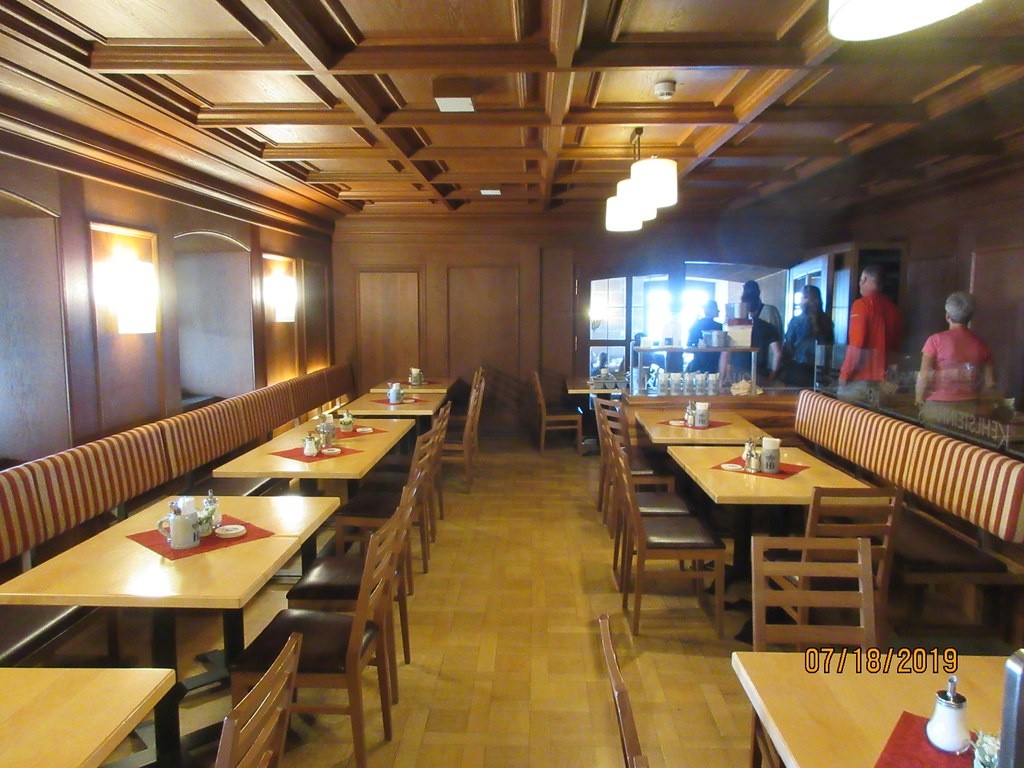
[631,158,678,208]
[605,197,642,232]
[616,178,656,221]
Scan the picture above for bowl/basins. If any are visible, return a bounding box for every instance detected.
[725,303,750,320]
[701,330,728,348]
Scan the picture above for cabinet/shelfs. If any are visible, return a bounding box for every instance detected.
[801,239,909,372]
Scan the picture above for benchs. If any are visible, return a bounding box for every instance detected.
[793,389,1024,641]
[0,362,356,669]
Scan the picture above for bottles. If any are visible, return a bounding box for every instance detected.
[742,437,756,460]
[745,453,751,470]
[407,367,425,386]
[304,433,317,456]
[686,411,693,427]
[685,405,694,421]
[926,675,971,753]
[750,453,759,473]
[316,413,336,449]
[344,410,350,419]
[204,489,221,528]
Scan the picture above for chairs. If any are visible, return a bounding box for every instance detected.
[142,367,906,768]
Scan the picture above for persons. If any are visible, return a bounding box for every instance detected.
[590,280,835,392]
[837,264,906,403]
[914,292,995,426]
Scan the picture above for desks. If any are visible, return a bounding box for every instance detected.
[564,375,623,456]
[729,651,1010,768]
[338,393,446,455]
[666,446,891,645]
[633,408,774,502]
[0,667,176,768]
[0,495,340,768]
[212,418,415,577]
[369,377,460,436]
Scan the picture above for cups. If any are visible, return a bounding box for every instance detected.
[657,379,718,396]
[158,513,200,550]
[761,447,780,473]
[412,374,421,385]
[695,410,710,427]
[387,388,401,404]
